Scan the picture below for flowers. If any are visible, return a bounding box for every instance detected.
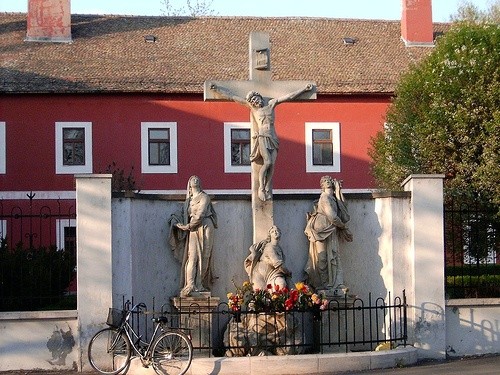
[225,276,329,321]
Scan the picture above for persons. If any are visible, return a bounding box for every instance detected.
[173,173,217,298]
[211,83,313,201]
[248,224,287,292]
[309,176,351,291]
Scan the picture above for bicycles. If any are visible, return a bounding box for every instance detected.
[88,294,193,375]
